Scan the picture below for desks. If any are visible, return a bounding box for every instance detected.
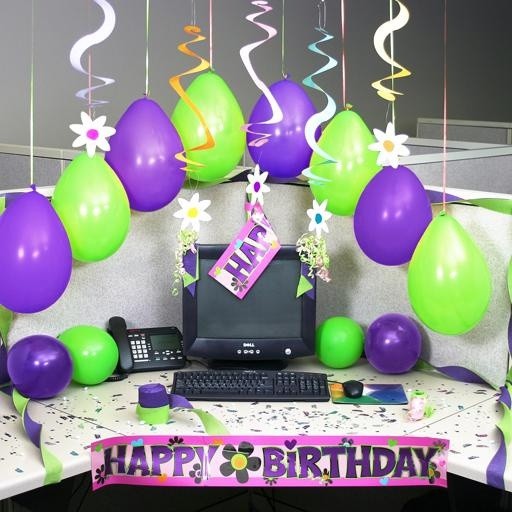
[0,352,511,503]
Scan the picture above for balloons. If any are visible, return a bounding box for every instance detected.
[0,184,71,314]
[7,334,74,400]
[170,73,246,182]
[308,104,383,216]
[57,325,120,385]
[247,74,321,178]
[52,146,131,263]
[363,313,422,374]
[105,92,187,212]
[316,316,364,369]
[354,165,432,266]
[407,210,491,335]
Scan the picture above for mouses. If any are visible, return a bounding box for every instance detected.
[343,380,364,398]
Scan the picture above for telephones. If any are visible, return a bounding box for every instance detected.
[106,316,185,373]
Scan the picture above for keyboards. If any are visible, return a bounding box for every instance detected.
[170,369,331,402]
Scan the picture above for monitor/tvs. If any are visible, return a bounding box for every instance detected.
[182,243,318,371]
